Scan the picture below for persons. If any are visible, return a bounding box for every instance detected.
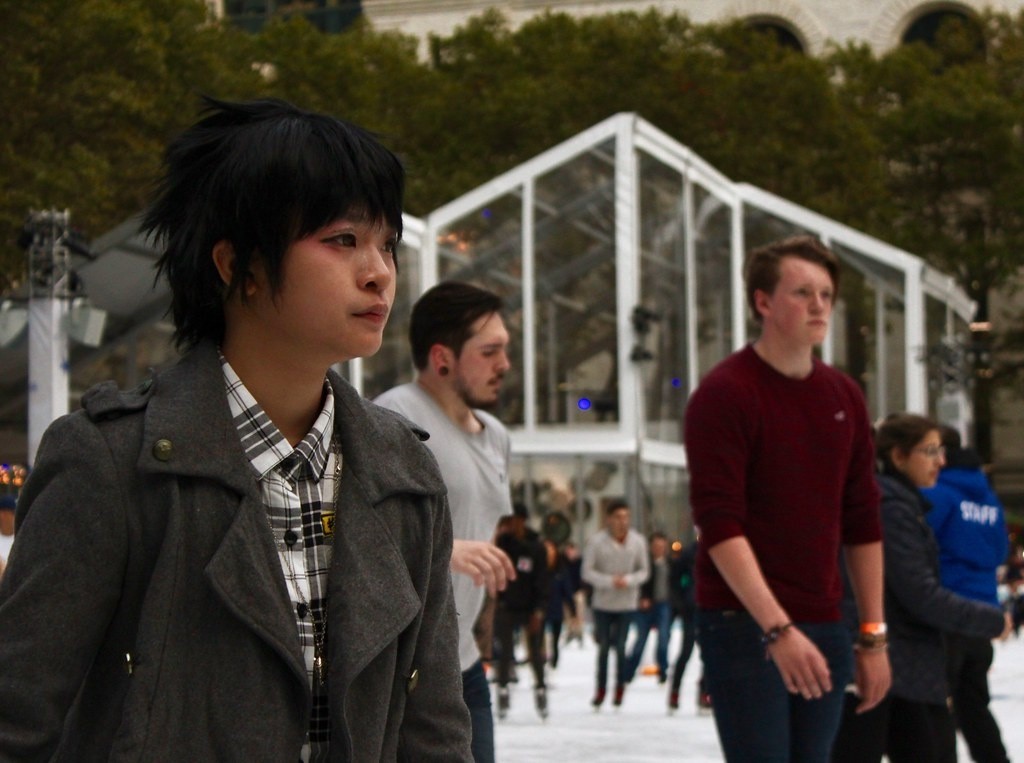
[372,280,516,763]
[830,412,1024,763]
[472,498,713,721]
[684,236,892,763]
[0,94,471,763]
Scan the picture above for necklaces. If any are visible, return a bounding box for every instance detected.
[266,431,341,686]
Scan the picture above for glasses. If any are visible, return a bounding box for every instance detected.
[915,446,945,459]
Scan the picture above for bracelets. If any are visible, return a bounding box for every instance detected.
[761,622,793,660]
[854,622,887,654]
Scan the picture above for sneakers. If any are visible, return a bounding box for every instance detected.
[670,688,679,709]
[498,688,509,716]
[533,687,548,722]
[613,685,624,706]
[696,687,713,715]
[592,684,606,705]
